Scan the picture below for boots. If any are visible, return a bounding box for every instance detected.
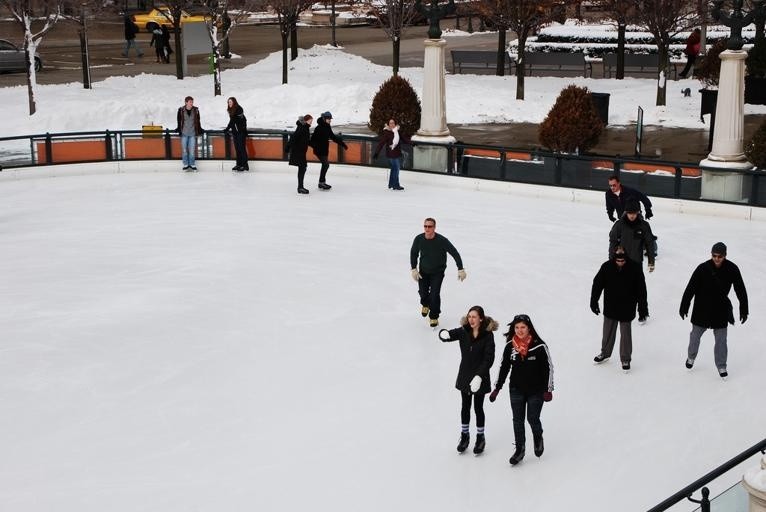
[510,441,525,464]
[457,432,469,451]
[473,434,485,453]
[534,432,544,456]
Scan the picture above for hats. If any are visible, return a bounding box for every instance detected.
[624,201,640,215]
[613,246,626,259]
[324,112,332,119]
[304,115,312,122]
[712,242,726,256]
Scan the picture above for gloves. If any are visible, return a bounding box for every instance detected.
[609,216,616,222]
[469,375,482,393]
[411,269,422,281]
[680,310,688,320]
[441,330,450,340]
[372,153,378,160]
[646,212,652,219]
[590,304,600,314]
[489,389,499,402]
[458,268,466,282]
[647,264,655,273]
[544,391,552,402]
[740,315,747,324]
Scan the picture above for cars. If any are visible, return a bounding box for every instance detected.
[131,6,213,32]
[0,38,42,75]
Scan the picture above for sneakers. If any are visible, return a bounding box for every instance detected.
[430,319,438,327]
[298,188,309,194]
[232,166,248,171]
[686,357,694,369]
[594,354,610,362]
[718,368,728,377]
[422,306,429,317]
[318,184,331,189]
[389,185,403,190]
[621,361,630,370]
[183,165,197,170]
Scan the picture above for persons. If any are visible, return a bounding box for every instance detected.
[678,28,700,79]
[310,112,348,189]
[439,306,495,457]
[605,176,652,222]
[286,115,315,194]
[679,242,748,377]
[178,96,202,169]
[121,13,144,57]
[590,247,649,370]
[489,315,554,464]
[223,98,249,171]
[150,23,171,64]
[609,200,657,272]
[373,117,416,190]
[410,218,466,326]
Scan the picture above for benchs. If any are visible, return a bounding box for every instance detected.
[602,54,671,78]
[525,52,592,76]
[450,51,517,74]
